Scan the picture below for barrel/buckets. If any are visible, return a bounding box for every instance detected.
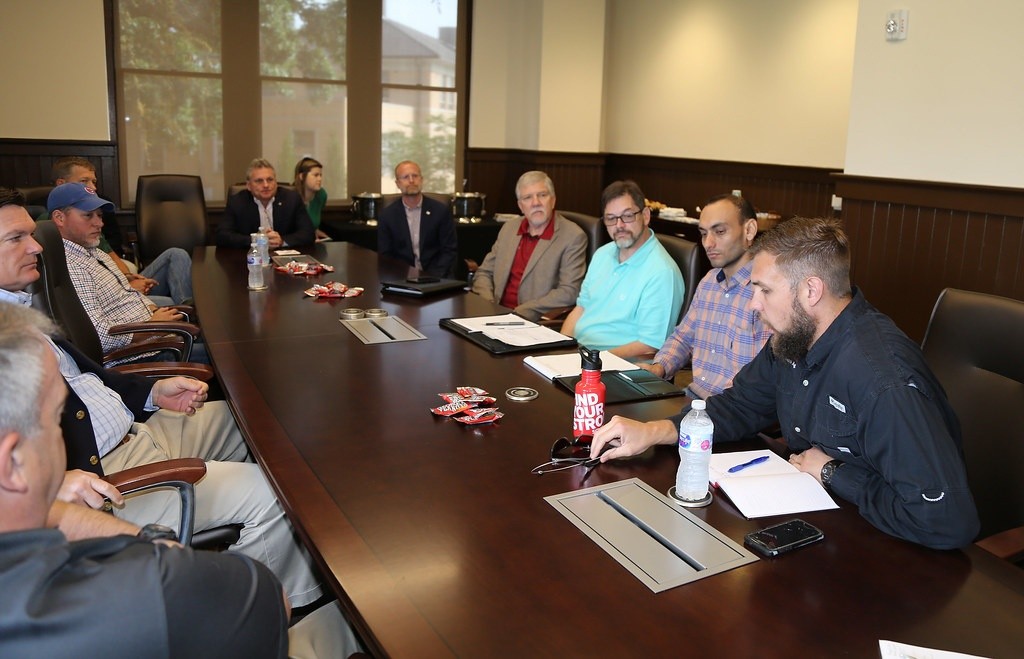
[351,195,383,222]
[449,196,484,223]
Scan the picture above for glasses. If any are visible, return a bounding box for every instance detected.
[550,435,617,485]
[601,209,641,225]
[530,453,603,473]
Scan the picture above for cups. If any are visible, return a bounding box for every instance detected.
[250,233,264,243]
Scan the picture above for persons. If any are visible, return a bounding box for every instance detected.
[35,156,209,368]
[0,189,363,659]
[472,170,588,321]
[0,301,293,659]
[215,159,316,248]
[294,157,328,240]
[561,181,685,365]
[590,218,981,550]
[376,160,457,279]
[633,195,773,400]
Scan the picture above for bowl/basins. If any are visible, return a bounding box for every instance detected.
[756,211,781,232]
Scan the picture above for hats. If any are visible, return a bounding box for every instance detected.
[47,181,114,212]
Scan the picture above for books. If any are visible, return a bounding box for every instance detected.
[380,277,468,296]
[447,313,538,333]
[708,450,839,518]
[524,350,641,380]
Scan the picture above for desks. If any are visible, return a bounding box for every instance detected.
[192,242,1024,659]
[320,218,504,265]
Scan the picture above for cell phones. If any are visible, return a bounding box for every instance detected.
[407,277,441,283]
[744,519,824,558]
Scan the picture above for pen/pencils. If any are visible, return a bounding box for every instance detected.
[728,455,769,472]
[486,322,524,325]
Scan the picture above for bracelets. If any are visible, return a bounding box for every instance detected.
[124,273,132,275]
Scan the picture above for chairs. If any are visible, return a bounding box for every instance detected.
[25,174,244,547]
[655,234,701,325]
[559,211,602,267]
[920,287,1024,564]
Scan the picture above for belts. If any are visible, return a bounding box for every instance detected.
[113,433,130,449]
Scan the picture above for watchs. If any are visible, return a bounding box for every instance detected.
[820,460,846,488]
[137,523,176,542]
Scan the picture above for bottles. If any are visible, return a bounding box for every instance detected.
[247,243,264,288]
[573,346,606,439]
[257,227,269,265]
[676,400,714,501]
